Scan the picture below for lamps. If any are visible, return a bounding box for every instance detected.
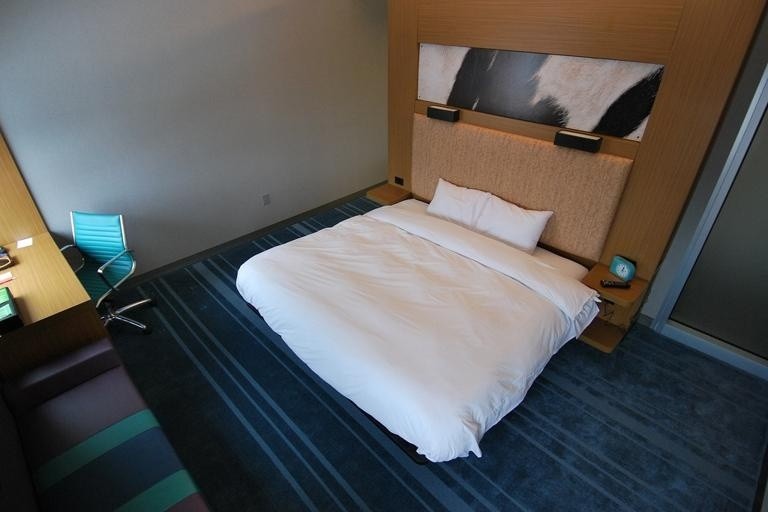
[427,104,460,122]
[554,128,603,153]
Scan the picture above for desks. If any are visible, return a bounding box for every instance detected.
[0,231,113,380]
[573,263,649,354]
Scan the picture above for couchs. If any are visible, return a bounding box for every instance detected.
[1,335,210,511]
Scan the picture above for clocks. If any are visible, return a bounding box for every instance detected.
[609,256,636,282]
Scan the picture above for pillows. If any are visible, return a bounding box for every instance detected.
[426,177,553,258]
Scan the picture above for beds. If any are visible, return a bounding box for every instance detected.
[235,113,634,465]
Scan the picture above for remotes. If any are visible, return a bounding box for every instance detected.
[601,279,631,289]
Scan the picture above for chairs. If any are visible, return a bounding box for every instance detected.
[59,211,158,335]
